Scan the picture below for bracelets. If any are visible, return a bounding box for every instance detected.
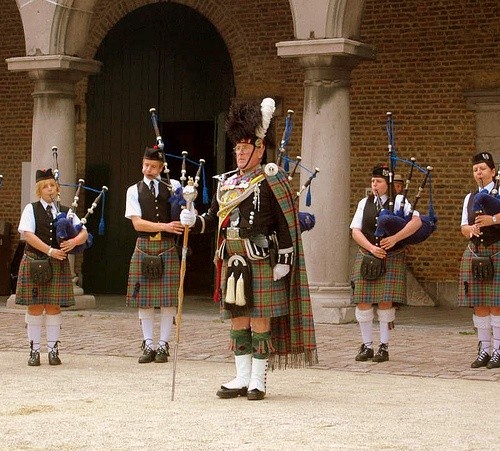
[48,247,54,256]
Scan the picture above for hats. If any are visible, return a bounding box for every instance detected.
[471,152,493,165]
[395,170,408,181]
[225,93,285,147]
[36,168,56,182]
[372,166,390,178]
[143,146,163,160]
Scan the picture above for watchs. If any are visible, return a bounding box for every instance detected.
[492,217,496,223]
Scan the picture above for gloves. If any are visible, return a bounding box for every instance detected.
[180,202,198,228]
[273,263,290,281]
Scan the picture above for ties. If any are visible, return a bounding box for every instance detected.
[377,198,382,208]
[150,180,155,196]
[46,205,53,219]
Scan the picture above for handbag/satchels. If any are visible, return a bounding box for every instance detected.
[472,257,494,281]
[29,259,52,285]
[142,255,163,279]
[360,255,385,281]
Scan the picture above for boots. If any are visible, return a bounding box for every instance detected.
[216,353,254,398]
[248,356,270,399]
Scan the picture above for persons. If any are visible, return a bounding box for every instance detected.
[180,144,320,400]
[125,148,191,363]
[15,167,88,366]
[349,167,422,362]
[394,174,405,195]
[458,152,500,369]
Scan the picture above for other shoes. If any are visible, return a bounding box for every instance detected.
[373,342,389,362]
[49,341,61,364]
[486,346,500,368]
[138,338,156,363]
[471,340,492,367]
[28,340,40,365]
[355,341,374,361]
[155,340,170,363]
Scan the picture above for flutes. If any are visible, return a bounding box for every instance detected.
[375,111,437,253]
[277,109,320,233]
[149,107,206,222]
[470,164,500,248]
[48,145,109,253]
